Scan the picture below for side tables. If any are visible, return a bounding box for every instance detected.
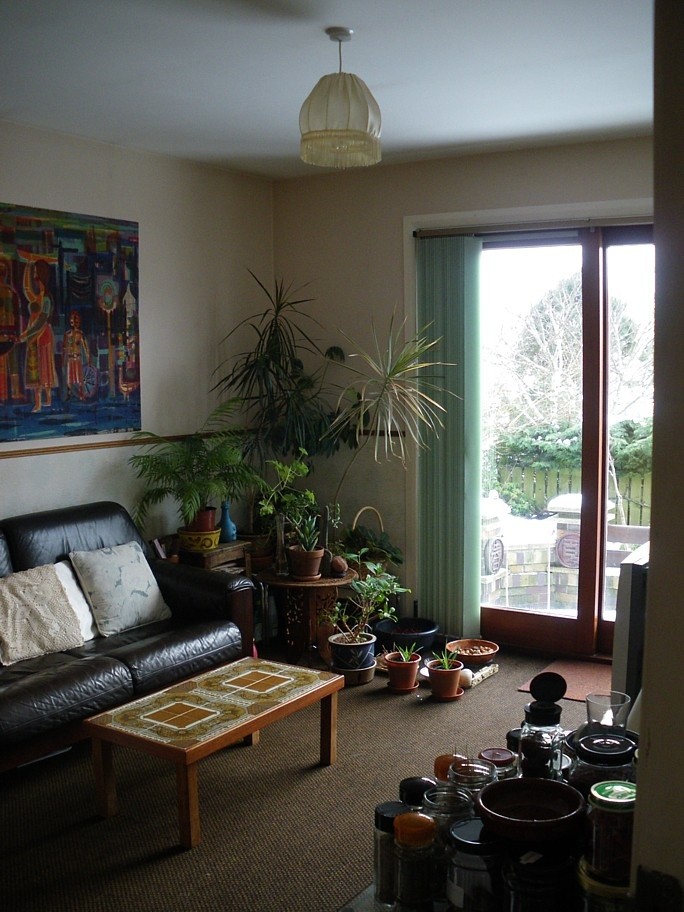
[173,540,252,579]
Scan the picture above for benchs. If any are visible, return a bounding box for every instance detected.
[0,501,256,774]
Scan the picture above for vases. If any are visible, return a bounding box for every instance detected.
[447,638,499,663]
[377,616,439,653]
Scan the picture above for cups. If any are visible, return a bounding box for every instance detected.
[585,689,631,729]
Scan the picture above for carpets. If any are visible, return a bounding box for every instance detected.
[518,658,611,701]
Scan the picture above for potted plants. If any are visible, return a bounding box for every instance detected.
[328,561,412,669]
[428,648,464,696]
[128,266,464,579]
[385,642,424,688]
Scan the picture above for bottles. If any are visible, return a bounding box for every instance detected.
[374,672,640,911]
[215,501,236,543]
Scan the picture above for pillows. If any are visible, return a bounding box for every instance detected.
[0,560,99,666]
[69,540,171,638]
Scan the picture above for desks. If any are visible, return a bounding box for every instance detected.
[83,656,344,848]
[260,568,357,665]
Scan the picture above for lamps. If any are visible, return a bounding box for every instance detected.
[298,27,382,167]
